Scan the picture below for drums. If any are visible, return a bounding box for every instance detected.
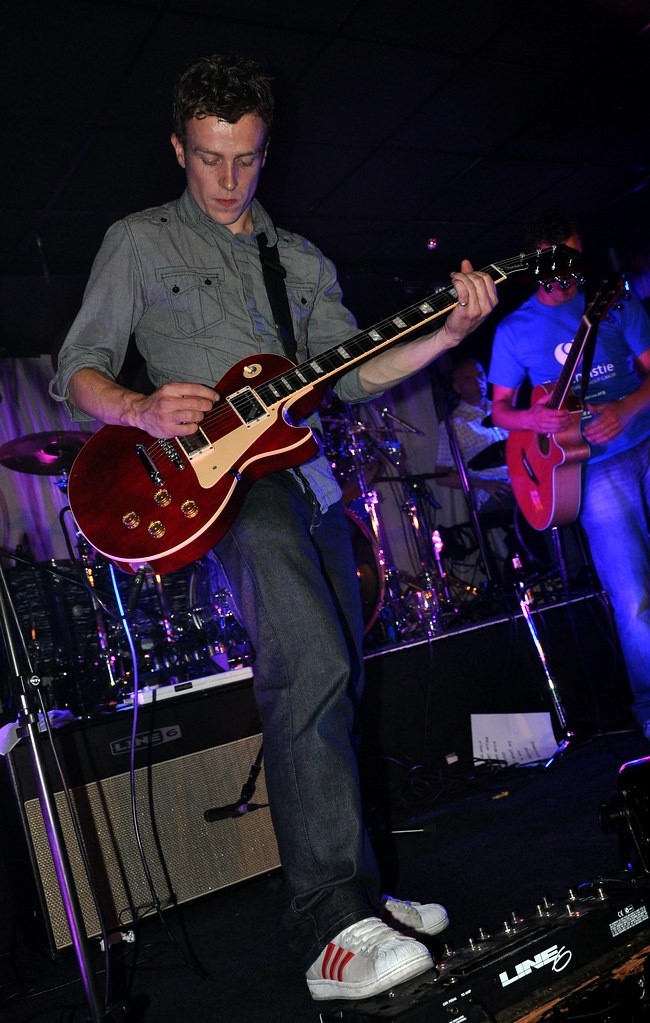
[188,504,386,664]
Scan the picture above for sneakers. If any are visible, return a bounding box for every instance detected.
[304,917,435,1001]
[376,896,449,939]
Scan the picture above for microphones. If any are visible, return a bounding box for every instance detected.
[204,803,258,823]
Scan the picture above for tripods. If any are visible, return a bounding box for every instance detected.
[340,401,494,643]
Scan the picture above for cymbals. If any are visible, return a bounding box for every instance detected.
[0,429,95,476]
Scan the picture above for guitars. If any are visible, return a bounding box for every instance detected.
[505,264,630,532]
[67,240,589,576]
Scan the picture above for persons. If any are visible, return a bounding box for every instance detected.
[487,226,649,741]
[435,359,516,515]
[49,54,500,1000]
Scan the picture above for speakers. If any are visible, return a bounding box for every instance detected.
[0,676,284,963]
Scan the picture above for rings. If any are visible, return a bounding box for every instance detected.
[459,302,467,306]
